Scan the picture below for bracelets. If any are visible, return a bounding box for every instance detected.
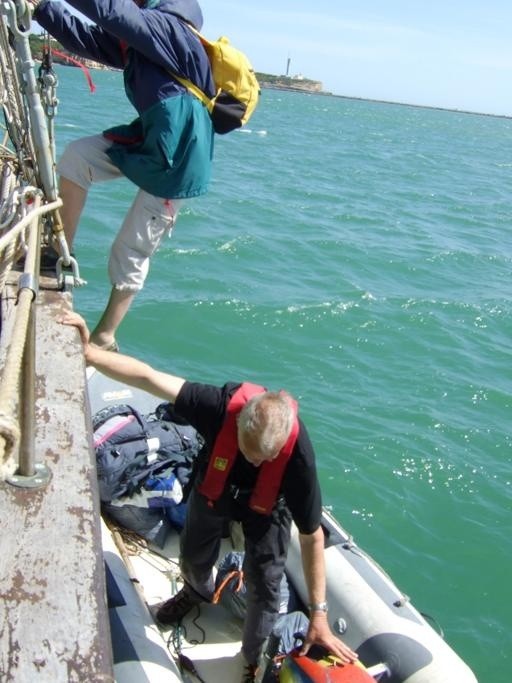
[305,598,330,612]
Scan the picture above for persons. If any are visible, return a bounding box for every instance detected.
[16,0,221,370]
[59,304,362,682]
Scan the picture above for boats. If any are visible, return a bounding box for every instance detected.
[86,360,479,682]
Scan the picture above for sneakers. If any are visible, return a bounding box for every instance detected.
[154,580,208,626]
[14,246,78,275]
[238,646,266,682]
[86,342,120,367]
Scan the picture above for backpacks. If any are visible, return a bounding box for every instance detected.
[163,13,261,135]
